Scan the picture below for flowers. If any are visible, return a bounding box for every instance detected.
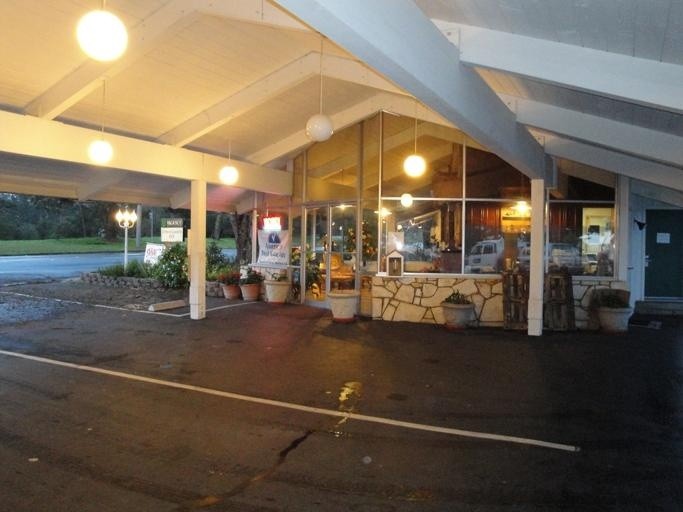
[216,267,264,285]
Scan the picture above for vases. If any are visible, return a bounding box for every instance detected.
[219,283,260,301]
[326,288,360,323]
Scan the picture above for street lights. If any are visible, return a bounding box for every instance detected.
[115,210,137,274]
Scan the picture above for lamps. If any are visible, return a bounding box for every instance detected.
[304,38,333,143]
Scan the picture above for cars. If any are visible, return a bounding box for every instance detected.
[469,238,585,275]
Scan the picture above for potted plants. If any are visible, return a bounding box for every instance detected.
[587,290,633,336]
[439,289,475,333]
[262,270,292,305]
